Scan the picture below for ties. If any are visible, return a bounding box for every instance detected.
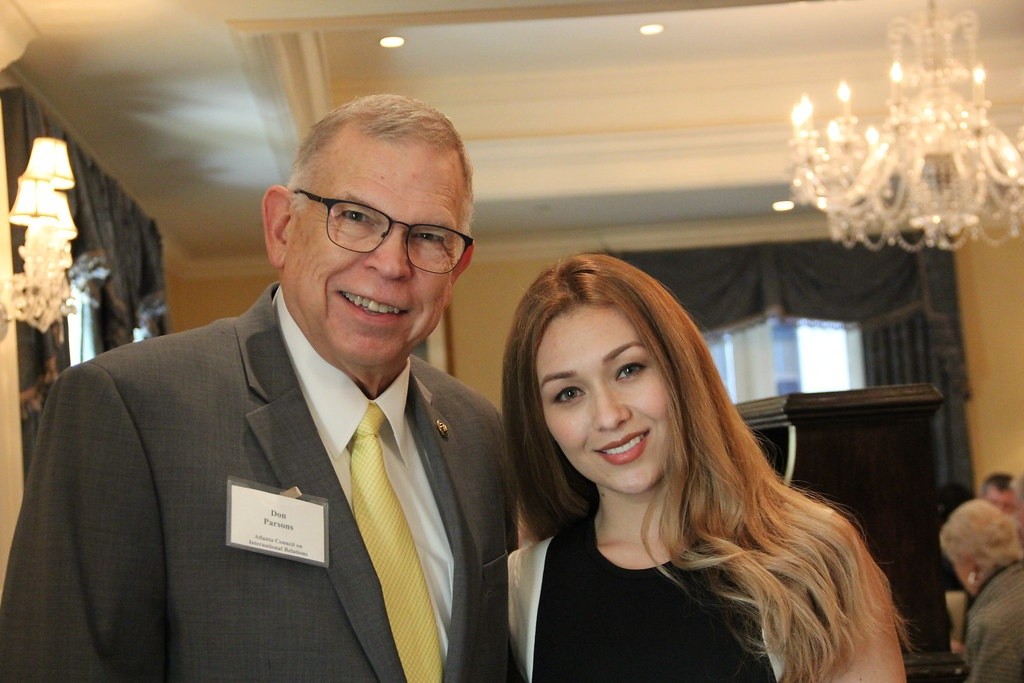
[347,403,443,683]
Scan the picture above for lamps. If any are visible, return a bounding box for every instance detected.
[780,1,1024,253]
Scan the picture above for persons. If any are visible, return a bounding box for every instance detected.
[0,95,518,683]
[937,474,1024,683]
[508,256,908,682]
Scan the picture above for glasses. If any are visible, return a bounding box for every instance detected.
[293,188,473,274]
